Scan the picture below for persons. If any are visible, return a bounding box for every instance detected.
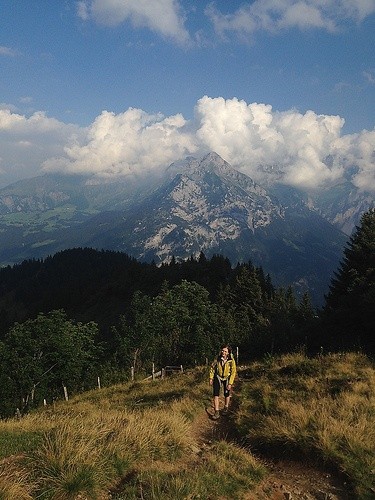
[206,345,237,420]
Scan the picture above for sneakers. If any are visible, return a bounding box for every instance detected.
[211,411,221,420]
[220,406,229,416]
